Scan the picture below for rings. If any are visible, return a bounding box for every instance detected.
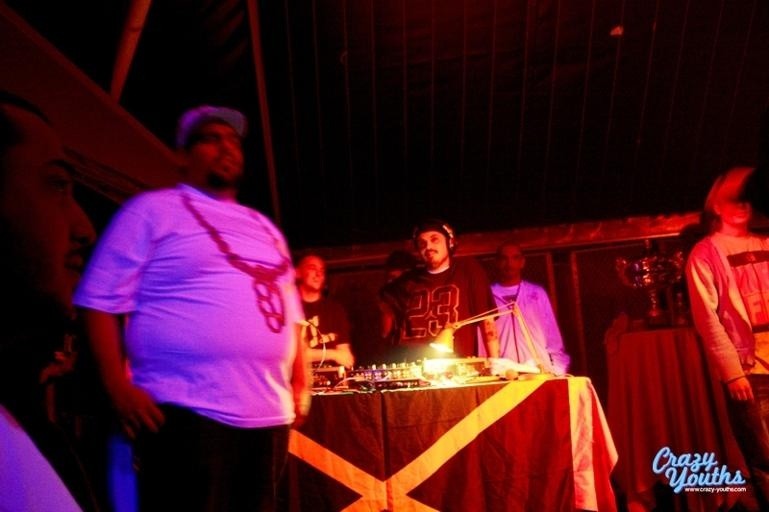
[124,428,132,435]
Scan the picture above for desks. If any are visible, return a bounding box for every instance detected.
[602,328,761,512]
[287,377,620,512]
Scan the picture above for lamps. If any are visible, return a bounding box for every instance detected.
[429,301,556,380]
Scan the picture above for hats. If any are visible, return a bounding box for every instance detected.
[176,105,246,143]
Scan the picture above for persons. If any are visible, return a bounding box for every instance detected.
[72,102,310,512]
[366,216,501,358]
[357,249,418,366]
[0,90,98,512]
[476,239,572,377]
[682,164,769,507]
[290,254,357,389]
[41,308,120,512]
